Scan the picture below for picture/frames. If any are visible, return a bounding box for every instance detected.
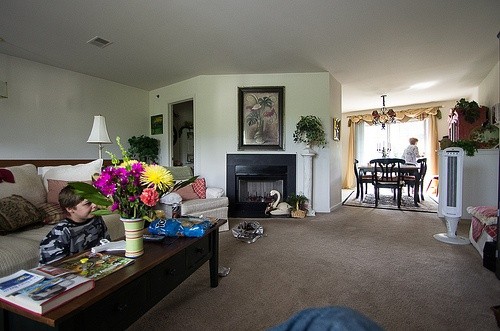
[237,86,285,151]
[333,117,342,141]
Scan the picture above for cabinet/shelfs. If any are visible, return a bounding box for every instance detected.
[435,105,499,219]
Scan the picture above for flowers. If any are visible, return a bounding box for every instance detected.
[67,134,200,222]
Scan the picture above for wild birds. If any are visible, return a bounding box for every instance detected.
[264,190,295,217]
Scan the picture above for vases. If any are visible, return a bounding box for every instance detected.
[120,218,145,258]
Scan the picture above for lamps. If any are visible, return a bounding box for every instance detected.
[86,114,112,158]
[372,95,396,130]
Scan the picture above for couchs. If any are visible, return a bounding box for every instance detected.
[153,166,229,233]
[465,206,500,258]
[0,159,125,279]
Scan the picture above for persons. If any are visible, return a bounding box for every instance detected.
[402,137,425,177]
[36,185,113,267]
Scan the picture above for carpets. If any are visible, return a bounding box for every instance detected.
[342,185,439,213]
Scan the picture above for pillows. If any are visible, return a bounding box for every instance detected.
[0,158,103,236]
[169,178,206,199]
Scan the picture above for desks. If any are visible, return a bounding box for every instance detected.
[358,164,420,207]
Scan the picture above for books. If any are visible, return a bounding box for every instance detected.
[0,263,96,318]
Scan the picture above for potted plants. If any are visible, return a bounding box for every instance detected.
[293,115,326,150]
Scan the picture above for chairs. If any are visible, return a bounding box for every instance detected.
[354,157,429,208]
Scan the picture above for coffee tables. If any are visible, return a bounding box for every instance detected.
[0,216,227,331]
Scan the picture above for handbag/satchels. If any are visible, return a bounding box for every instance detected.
[232,220,264,243]
[148,217,212,237]
